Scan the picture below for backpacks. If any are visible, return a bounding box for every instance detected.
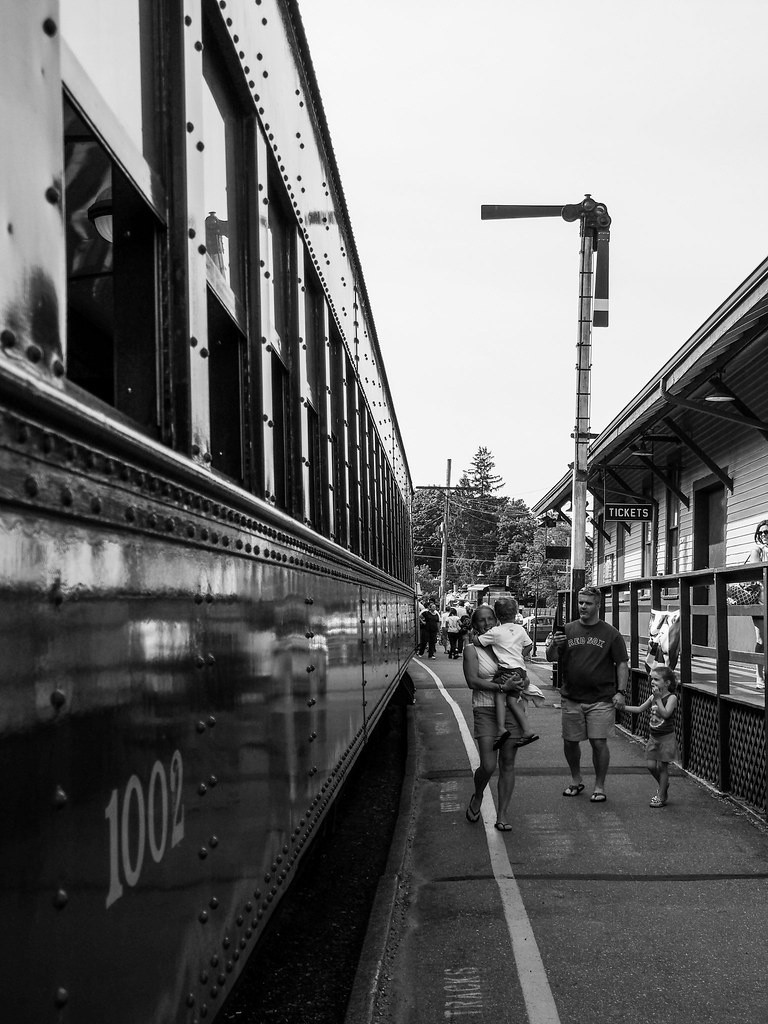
[461,615,472,630]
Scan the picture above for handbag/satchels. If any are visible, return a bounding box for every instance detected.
[726,546,763,604]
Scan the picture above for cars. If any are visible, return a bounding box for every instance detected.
[521,616,554,643]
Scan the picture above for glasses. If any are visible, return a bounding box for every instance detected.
[756,529,768,535]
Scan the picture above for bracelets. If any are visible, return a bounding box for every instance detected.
[499,683,503,694]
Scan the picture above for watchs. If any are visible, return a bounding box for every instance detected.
[617,689,628,696]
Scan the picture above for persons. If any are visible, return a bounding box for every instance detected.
[462,605,530,833]
[240,606,331,747]
[750,519,767,690]
[416,598,525,661]
[545,587,629,803]
[471,597,539,750]
[613,666,678,808]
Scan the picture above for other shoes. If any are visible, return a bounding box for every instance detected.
[417,647,463,660]
[755,679,765,689]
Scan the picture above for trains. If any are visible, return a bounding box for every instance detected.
[0,1,424,1024]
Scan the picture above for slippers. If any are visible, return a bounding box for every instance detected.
[493,731,511,751]
[563,783,585,796]
[649,783,670,807]
[494,822,513,831]
[466,793,482,823]
[590,792,606,802]
[513,733,540,747]
